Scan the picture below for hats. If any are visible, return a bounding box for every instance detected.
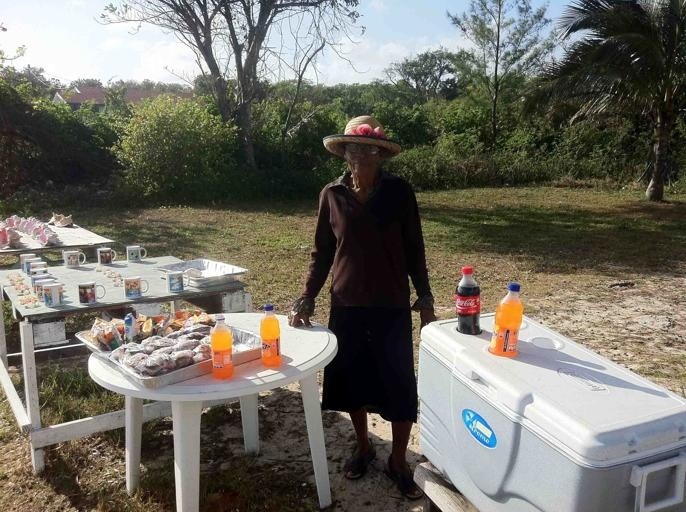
[322,114,402,158]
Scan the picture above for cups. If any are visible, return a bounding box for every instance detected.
[20,246,190,307]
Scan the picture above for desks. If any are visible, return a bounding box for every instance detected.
[413,463,480,512]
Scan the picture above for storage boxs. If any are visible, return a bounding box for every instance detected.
[417,307,686,512]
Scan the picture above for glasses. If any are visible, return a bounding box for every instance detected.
[343,142,379,154]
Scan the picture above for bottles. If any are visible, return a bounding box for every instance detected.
[209,315,235,379]
[456,266,524,356]
[259,305,282,370]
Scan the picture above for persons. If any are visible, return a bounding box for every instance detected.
[288,115,439,501]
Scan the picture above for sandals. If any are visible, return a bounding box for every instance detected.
[344,437,376,479]
[385,454,424,499]
